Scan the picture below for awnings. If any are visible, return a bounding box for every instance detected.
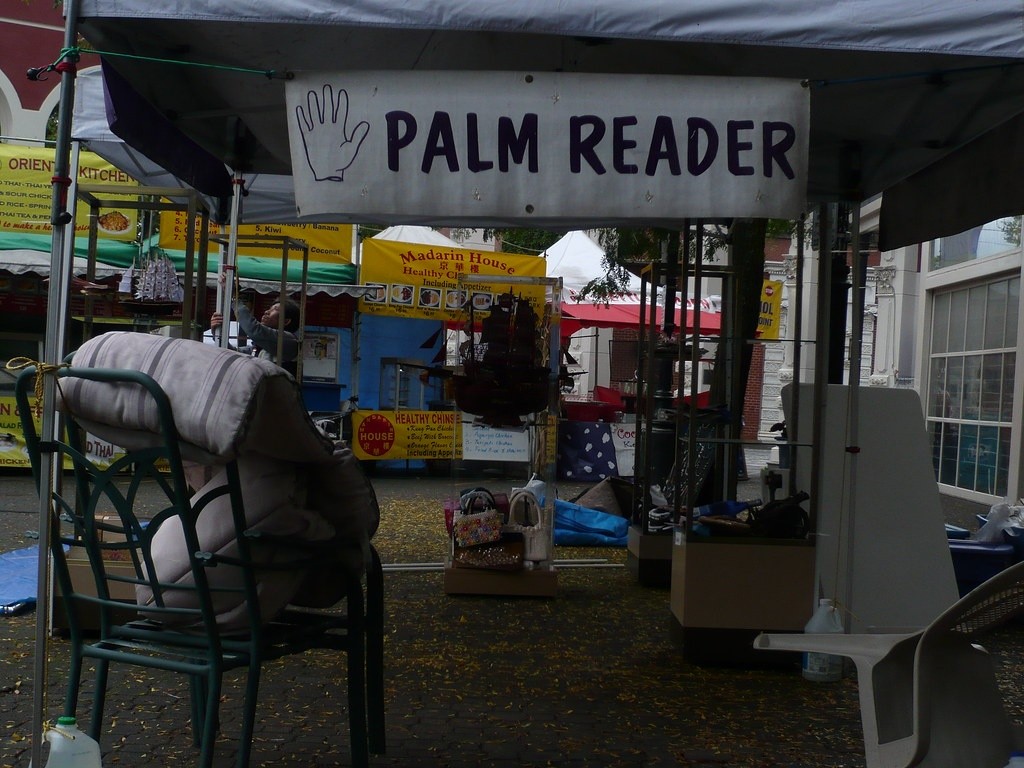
[0,249,383,301]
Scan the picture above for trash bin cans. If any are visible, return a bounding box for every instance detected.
[946,523,1014,601]
[976,512,1024,561]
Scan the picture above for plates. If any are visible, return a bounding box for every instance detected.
[421,290,440,306]
[97,213,132,233]
[472,294,492,309]
[392,285,412,302]
[446,292,467,307]
[365,285,385,302]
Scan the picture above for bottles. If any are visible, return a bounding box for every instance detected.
[694,499,763,521]
[1004,750,1024,768]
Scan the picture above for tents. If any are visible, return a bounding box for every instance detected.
[31,0,1023,768]
[358,225,764,344]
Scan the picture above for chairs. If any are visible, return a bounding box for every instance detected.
[753,561,1024,768]
[15,362,386,768]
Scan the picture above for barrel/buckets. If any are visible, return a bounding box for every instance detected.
[803,599,844,682]
[28,716,103,768]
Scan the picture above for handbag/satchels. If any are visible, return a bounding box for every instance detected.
[501,489,553,561]
[450,487,502,547]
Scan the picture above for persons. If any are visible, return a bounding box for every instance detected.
[210,271,300,380]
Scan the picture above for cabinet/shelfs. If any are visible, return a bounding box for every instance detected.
[624,200,834,669]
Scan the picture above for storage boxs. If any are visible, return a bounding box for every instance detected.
[444,568,557,596]
[944,523,970,539]
[53,512,153,631]
[948,539,1014,582]
